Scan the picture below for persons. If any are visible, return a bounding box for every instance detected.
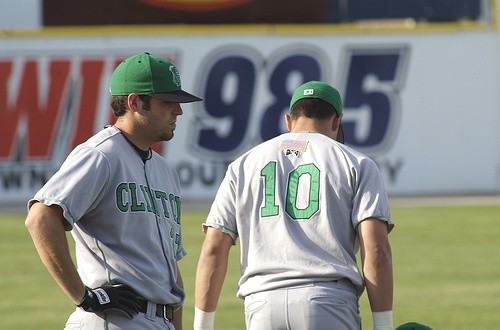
[25,52,204,330]
[193,81,395,330]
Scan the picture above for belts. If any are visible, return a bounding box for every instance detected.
[139,299,173,322]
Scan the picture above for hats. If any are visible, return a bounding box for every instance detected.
[290,80,344,144]
[109,52,203,103]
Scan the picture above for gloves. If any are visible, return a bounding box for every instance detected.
[76,280,143,319]
